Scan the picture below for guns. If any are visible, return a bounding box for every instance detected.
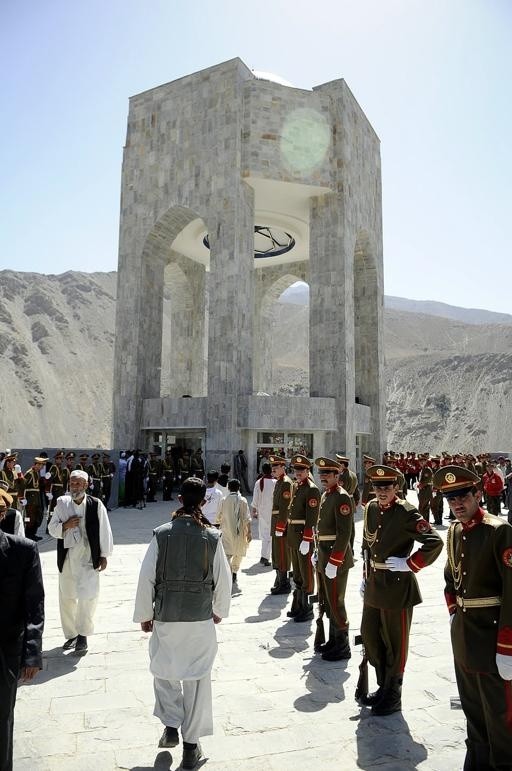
[353,549,372,699]
[309,527,326,651]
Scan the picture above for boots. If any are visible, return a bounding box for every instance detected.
[272,577,401,716]
[25,526,42,541]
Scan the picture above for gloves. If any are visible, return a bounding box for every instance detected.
[495,654,512,681]
[45,472,51,479]
[275,530,283,536]
[89,484,94,490]
[386,556,412,572]
[46,492,54,500]
[324,562,337,580]
[299,540,310,556]
[14,464,22,474]
[311,552,318,569]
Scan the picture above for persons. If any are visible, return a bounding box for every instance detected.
[363,451,511,559]
[354,463,444,714]
[199,453,360,658]
[0,450,210,771]
[433,463,511,771]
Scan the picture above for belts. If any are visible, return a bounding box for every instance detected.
[288,518,306,523]
[102,474,110,477]
[52,483,63,486]
[93,478,100,481]
[455,595,503,608]
[370,559,385,570]
[271,510,279,515]
[8,492,18,496]
[25,489,40,492]
[318,534,336,542]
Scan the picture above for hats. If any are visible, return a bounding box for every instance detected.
[53,451,110,460]
[70,469,88,482]
[34,457,50,464]
[267,452,512,495]
[0,480,9,492]
[4,452,18,461]
[0,488,14,511]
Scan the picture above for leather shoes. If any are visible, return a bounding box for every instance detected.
[182,744,200,769]
[64,636,77,649]
[158,728,179,748]
[76,634,87,651]
